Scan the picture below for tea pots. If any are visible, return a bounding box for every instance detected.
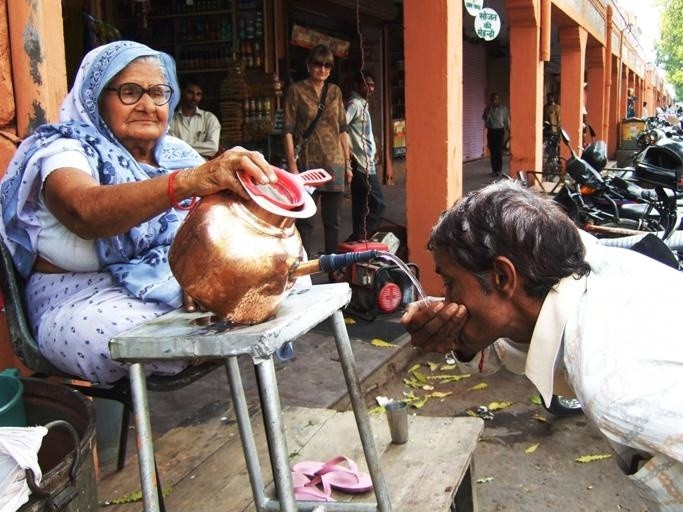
[168,163,379,326]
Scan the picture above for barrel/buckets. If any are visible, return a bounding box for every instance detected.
[0,376,101,512]
[0,367,27,427]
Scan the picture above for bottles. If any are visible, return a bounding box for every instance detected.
[245,95,271,125]
[221,9,262,38]
[180,39,260,69]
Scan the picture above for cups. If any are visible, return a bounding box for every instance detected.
[385,401,408,443]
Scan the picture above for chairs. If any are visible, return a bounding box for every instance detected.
[0,239,224,512]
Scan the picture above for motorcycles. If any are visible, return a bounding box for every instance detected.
[551,124,682,242]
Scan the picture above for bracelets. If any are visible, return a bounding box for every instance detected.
[346,165,353,171]
[345,158,353,163]
[169,171,196,211]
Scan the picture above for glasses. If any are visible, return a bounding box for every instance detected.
[103,82,174,106]
[313,60,331,68]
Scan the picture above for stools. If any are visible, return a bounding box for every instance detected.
[109,283,397,512]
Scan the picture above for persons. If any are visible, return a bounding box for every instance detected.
[169,80,222,159]
[63,3,122,92]
[282,45,353,260]
[1,38,312,383]
[627,88,635,117]
[543,92,560,148]
[400,178,683,512]
[642,102,649,118]
[345,74,385,242]
[482,93,510,177]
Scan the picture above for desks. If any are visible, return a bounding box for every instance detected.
[99,399,484,512]
